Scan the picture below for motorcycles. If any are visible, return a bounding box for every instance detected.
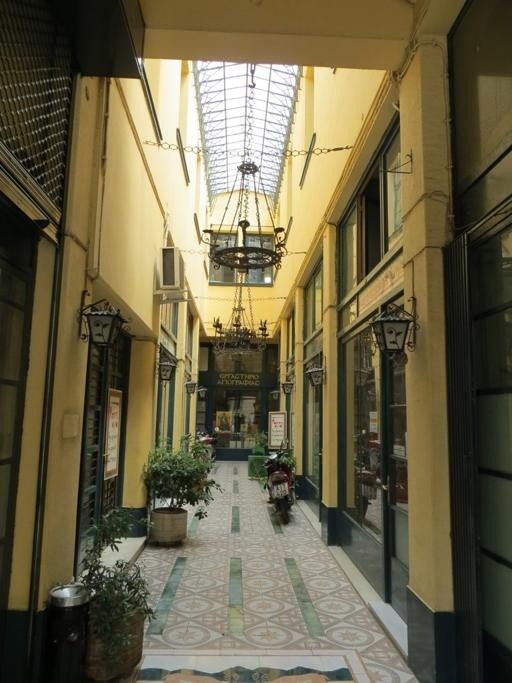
[194,430,219,463]
[351,455,378,524]
[259,438,293,522]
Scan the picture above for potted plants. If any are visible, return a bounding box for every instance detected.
[142,436,225,547]
[80,501,157,680]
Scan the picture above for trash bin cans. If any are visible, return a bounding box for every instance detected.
[45,584,90,683]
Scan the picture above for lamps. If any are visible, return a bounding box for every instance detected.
[184,377,197,395]
[303,354,326,387]
[280,375,296,394]
[366,297,423,360]
[77,288,131,353]
[154,352,177,382]
[211,268,268,356]
[202,58,291,275]
[271,388,281,399]
[198,386,208,397]
